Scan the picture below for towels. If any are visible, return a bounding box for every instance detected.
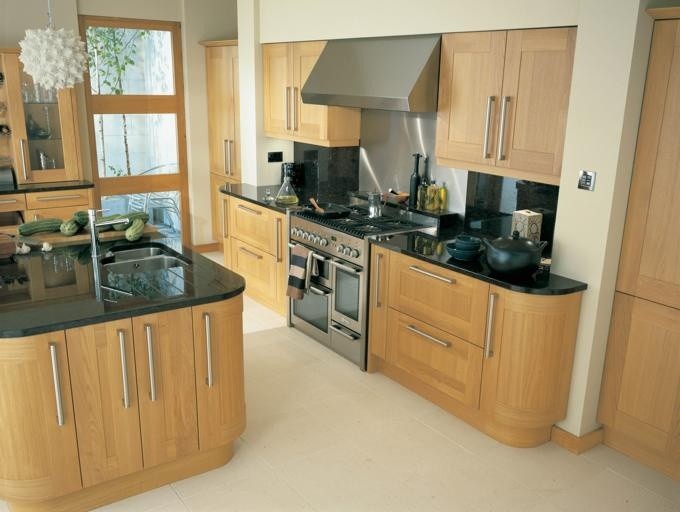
[286,245,319,300]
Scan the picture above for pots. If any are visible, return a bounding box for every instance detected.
[482,230,548,282]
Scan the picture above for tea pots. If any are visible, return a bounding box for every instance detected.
[366,191,387,219]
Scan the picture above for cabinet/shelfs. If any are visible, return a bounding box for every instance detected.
[65,307,199,511]
[262,38,361,148]
[434,27,580,186]
[3,182,98,226]
[0,330,83,511]
[387,246,490,413]
[192,292,246,475]
[1,251,96,314]
[370,243,391,363]
[478,281,583,449]
[210,173,239,252]
[218,191,289,324]
[599,7,680,489]
[4,48,85,191]
[199,39,241,179]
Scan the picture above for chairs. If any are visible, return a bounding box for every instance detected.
[126,191,181,222]
[126,192,178,234]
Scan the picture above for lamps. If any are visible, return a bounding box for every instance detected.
[18,1,88,90]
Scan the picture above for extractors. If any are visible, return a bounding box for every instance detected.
[297,34,441,118]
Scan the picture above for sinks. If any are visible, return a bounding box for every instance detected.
[103,248,165,264]
[106,254,188,273]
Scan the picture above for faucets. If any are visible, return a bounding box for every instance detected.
[89,206,129,258]
[92,259,133,304]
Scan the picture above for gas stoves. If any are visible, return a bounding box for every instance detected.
[296,201,423,242]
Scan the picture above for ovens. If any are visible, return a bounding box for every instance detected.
[284,239,368,374]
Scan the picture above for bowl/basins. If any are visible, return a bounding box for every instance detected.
[455,243,481,251]
[445,242,486,262]
[456,235,481,244]
[394,192,410,203]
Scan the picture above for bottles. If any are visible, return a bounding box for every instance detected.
[273,175,299,208]
[416,177,428,211]
[426,180,440,210]
[367,193,382,218]
[409,153,423,207]
[438,183,448,209]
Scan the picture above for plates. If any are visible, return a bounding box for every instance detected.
[303,202,351,219]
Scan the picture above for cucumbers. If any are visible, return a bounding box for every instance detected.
[72,210,89,226]
[125,218,145,241]
[112,211,150,231]
[18,217,63,236]
[60,219,80,236]
[84,213,121,233]
